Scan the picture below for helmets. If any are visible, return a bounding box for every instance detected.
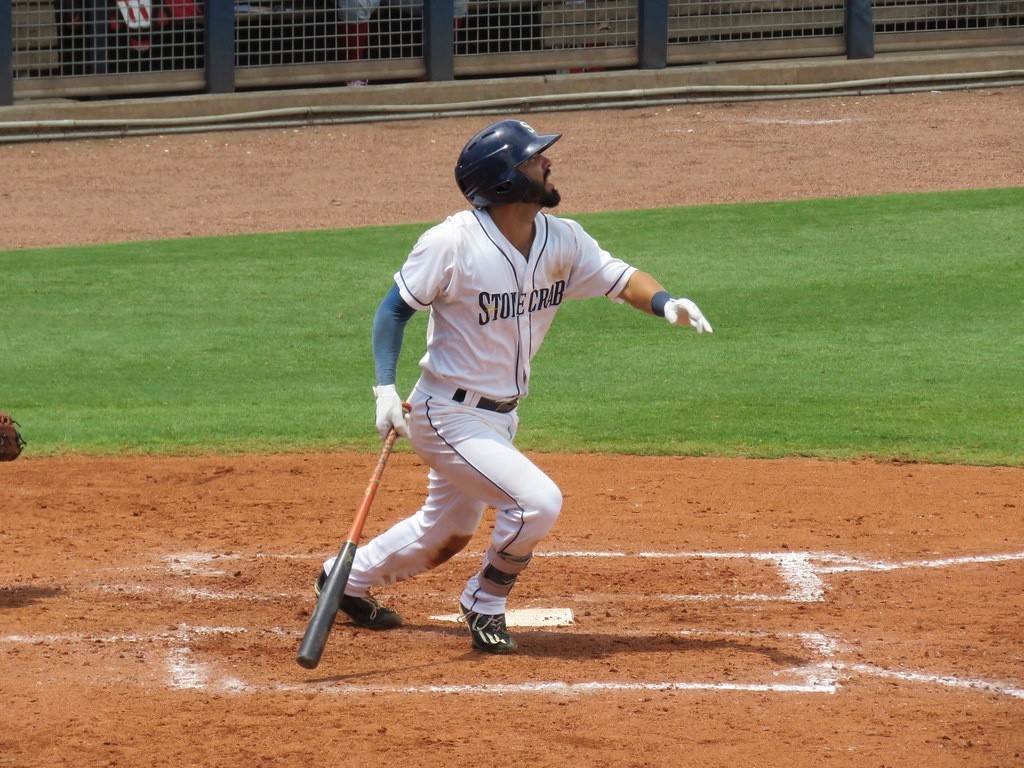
[455,119,562,208]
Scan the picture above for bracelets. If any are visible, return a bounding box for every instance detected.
[651,291,671,318]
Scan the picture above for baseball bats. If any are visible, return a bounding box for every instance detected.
[295,399,412,670]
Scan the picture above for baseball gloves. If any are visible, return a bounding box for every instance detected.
[0,411,28,463]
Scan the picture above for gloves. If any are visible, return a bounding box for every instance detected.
[650,293,713,335]
[374,385,411,444]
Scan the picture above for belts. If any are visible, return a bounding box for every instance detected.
[453,391,520,414]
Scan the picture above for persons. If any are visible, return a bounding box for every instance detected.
[338,0,467,86]
[315,119,714,655]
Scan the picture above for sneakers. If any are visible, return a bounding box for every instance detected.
[457,601,517,654]
[315,568,402,631]
